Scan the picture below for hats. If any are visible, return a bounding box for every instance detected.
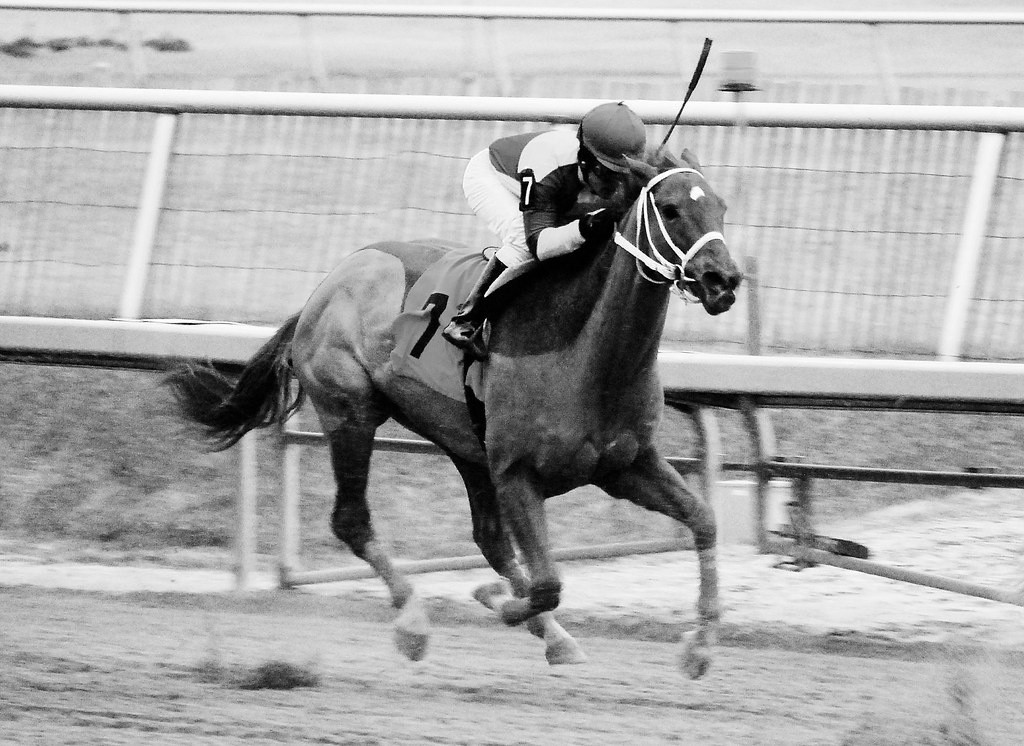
[581,101,646,173]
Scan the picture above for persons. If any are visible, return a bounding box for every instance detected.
[441,100,651,362]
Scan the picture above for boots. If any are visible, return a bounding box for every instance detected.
[440,251,510,362]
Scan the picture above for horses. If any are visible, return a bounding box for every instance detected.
[151,143,742,679]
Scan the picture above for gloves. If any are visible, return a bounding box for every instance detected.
[579,208,616,242]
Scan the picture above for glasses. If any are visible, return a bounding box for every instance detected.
[592,166,626,183]
